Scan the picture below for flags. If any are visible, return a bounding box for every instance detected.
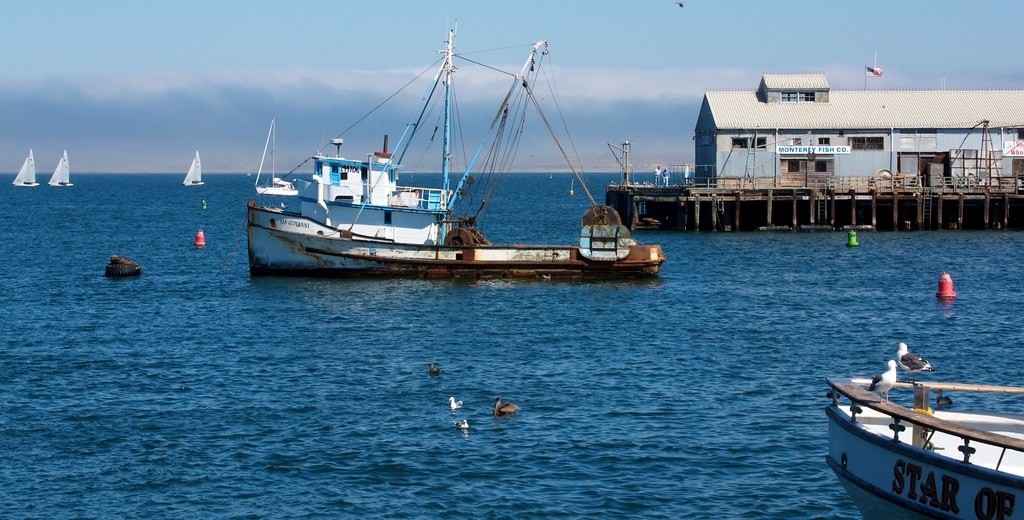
[866,66,883,77]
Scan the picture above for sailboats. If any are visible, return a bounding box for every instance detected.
[12,149,40,187]
[48,148,74,187]
[246,17,666,280]
[182,150,204,186]
[253,117,298,196]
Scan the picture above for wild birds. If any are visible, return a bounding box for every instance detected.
[867,342,938,405]
[674,1,684,8]
[427,363,521,428]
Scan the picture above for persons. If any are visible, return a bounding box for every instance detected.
[661,167,669,186]
[654,166,662,187]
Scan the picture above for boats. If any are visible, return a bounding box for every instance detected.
[825,376,1023,520]
